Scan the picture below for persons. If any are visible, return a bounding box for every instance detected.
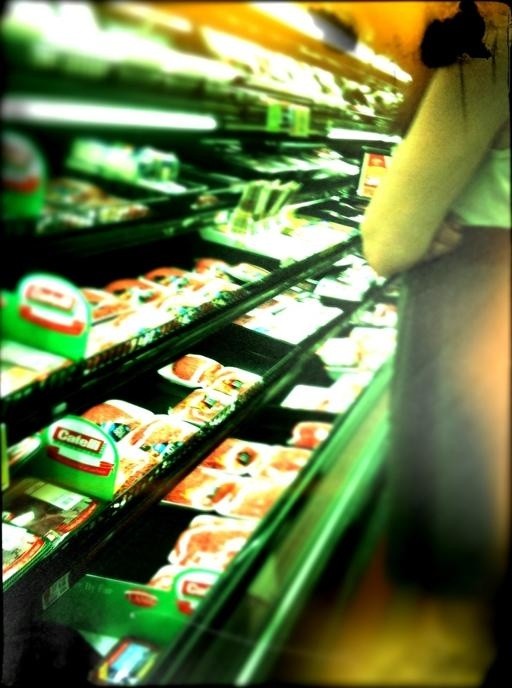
[358,0,512,687]
